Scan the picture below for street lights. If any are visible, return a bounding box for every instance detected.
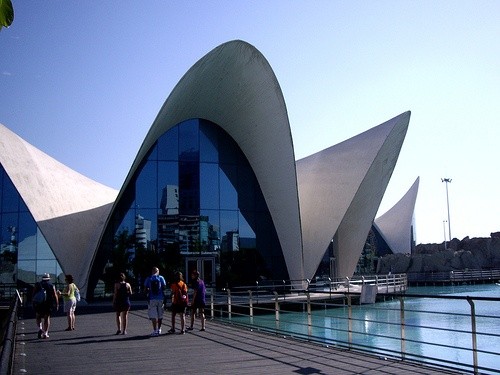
[443,219,448,250]
[440,178,453,250]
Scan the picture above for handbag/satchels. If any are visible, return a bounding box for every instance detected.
[75,288,81,301]
[31,282,46,303]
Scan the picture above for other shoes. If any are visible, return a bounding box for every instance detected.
[179,330,185,334]
[73,327,75,330]
[115,330,121,335]
[123,331,127,334]
[38,329,42,339]
[43,334,49,339]
[65,328,72,331]
[158,330,162,334]
[149,332,158,336]
[200,328,206,331]
[168,328,175,333]
[186,326,194,330]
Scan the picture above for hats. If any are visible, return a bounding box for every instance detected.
[42,273,50,280]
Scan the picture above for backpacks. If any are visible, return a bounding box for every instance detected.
[176,283,189,304]
[151,280,160,295]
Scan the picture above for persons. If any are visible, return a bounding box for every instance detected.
[32,273,59,339]
[168,271,188,334]
[187,270,205,330]
[112,273,132,335]
[144,267,166,336]
[57,275,79,331]
